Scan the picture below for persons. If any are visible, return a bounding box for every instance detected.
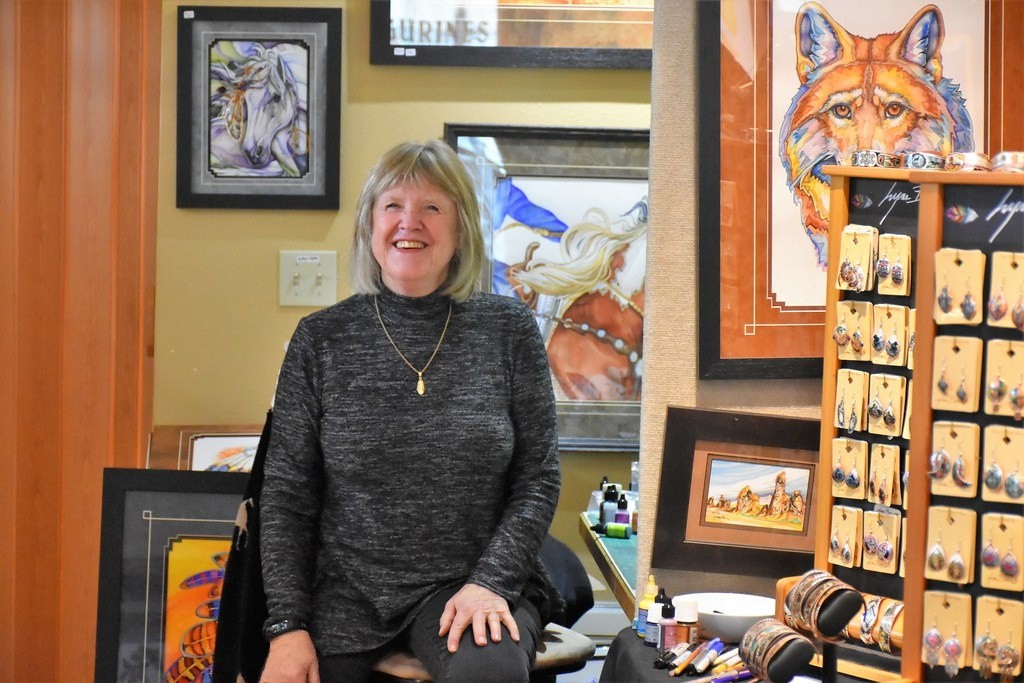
[258,140,560,683]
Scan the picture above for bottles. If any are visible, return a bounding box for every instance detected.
[631,575,699,655]
[593,460,639,539]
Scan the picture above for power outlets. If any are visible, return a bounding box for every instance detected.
[278,250,337,308]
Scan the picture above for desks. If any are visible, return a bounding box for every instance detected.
[598,625,874,683]
[579,512,638,623]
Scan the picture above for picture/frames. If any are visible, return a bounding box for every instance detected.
[176,5,342,211]
[443,124,651,454]
[697,0,1024,379]
[369,0,653,68]
[93,467,251,683]
[650,406,822,578]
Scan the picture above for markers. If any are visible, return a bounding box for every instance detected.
[652,637,761,683]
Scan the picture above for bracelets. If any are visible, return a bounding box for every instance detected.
[265,620,309,641]
[741,568,867,683]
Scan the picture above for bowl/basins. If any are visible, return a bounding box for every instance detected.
[672,593,789,645]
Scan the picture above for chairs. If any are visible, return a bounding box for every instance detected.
[210,409,596,683]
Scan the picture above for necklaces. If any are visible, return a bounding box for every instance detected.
[373,294,453,396]
